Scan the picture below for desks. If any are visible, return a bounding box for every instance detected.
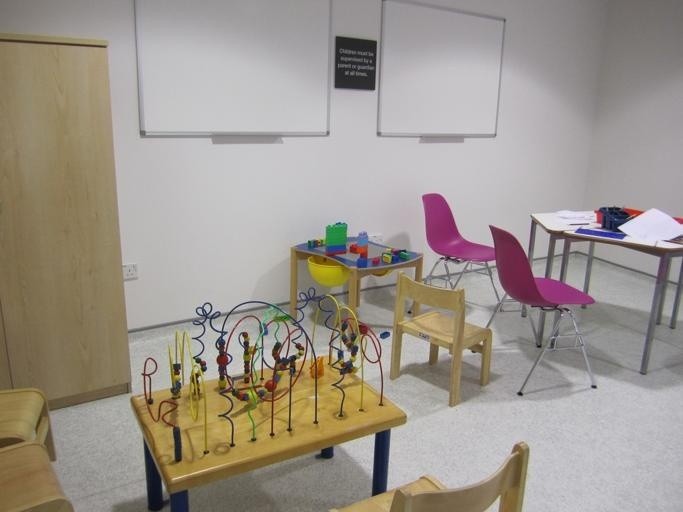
[522,210,683,376]
[289,238,423,326]
[130,355,410,512]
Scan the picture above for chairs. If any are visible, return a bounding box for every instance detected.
[331,441,530,512]
[423,192,504,312]
[0,388,59,462]
[0,440,74,512]
[390,270,494,408]
[483,224,598,396]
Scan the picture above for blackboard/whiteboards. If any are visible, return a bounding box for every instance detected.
[377,1,506,137]
[133,0,332,137]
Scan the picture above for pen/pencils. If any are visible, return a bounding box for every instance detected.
[606,203,624,212]
[569,223,589,226]
[626,214,637,220]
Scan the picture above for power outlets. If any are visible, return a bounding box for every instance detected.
[123,264,138,281]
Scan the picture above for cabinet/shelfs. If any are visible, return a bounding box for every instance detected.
[0,32,133,410]
